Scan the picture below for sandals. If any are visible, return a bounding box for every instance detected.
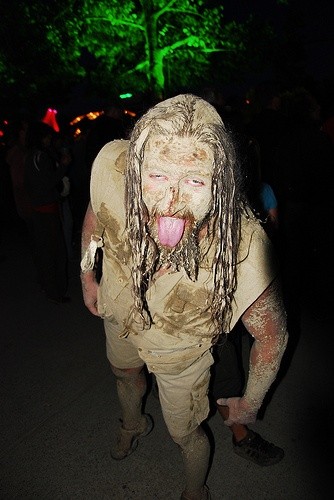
[110,413,153,460]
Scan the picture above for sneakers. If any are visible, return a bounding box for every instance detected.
[232,428,284,468]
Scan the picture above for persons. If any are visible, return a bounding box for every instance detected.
[200,79,334,465]
[5,108,32,222]
[22,120,71,306]
[80,93,289,500]
